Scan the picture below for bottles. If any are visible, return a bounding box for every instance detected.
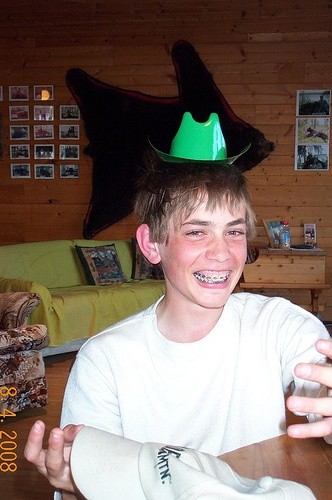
[278,220,290,250]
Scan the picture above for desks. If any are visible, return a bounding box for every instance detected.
[238,247,332,317]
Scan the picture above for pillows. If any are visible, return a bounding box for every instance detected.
[75,243,129,286]
[132,237,164,280]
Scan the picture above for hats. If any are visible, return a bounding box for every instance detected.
[71,426,316,499]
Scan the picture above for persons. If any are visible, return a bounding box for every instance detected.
[59,159,332,499]
[22,421,332,500]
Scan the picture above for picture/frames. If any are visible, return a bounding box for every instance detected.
[10,144,30,159]
[58,125,79,140]
[34,105,55,121]
[34,144,55,160]
[9,106,30,122]
[59,105,80,121]
[11,164,31,179]
[34,163,54,179]
[304,224,316,245]
[9,125,30,140]
[293,90,332,172]
[34,85,54,100]
[60,163,78,178]
[9,85,29,101]
[261,218,283,248]
[33,124,55,140]
[59,144,80,160]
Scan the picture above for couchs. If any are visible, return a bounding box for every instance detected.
[0,293,48,418]
[0,240,168,357]
